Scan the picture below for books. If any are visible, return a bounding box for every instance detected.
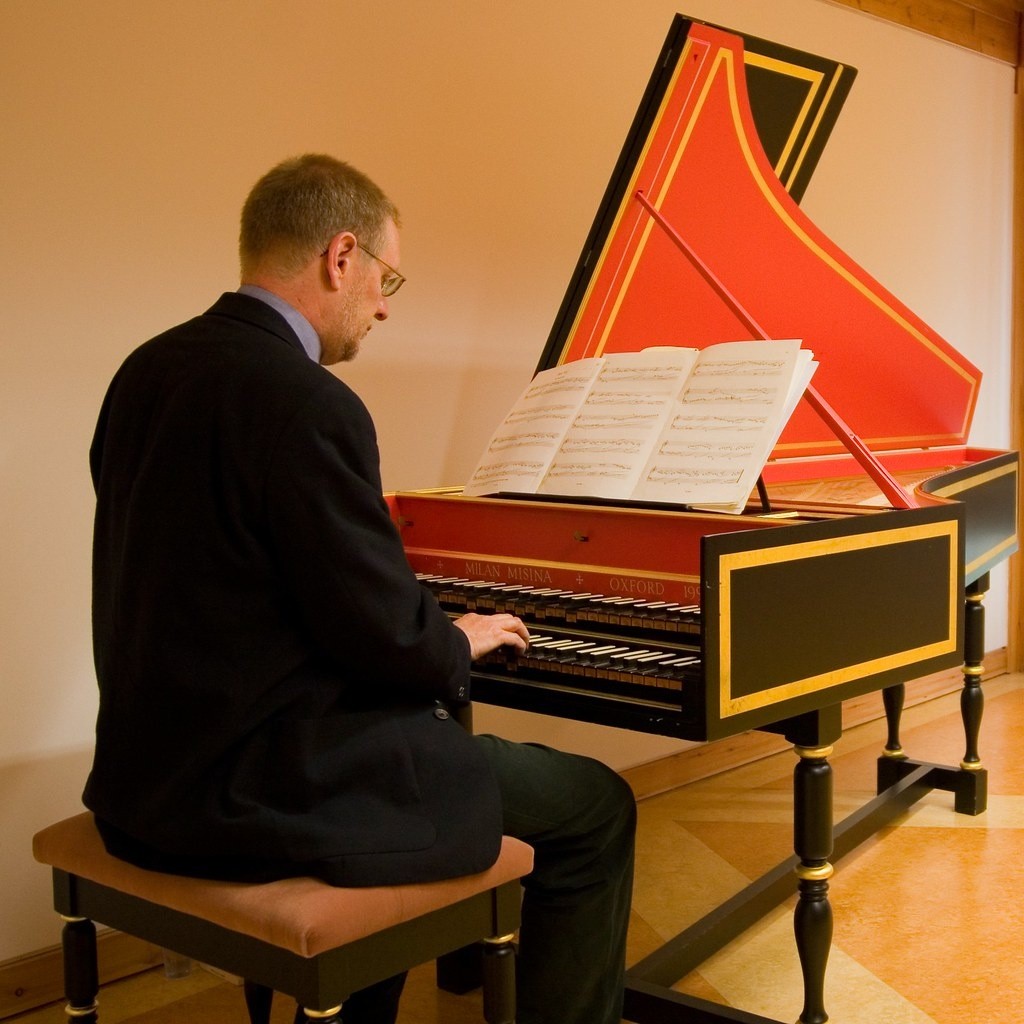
[456,337,823,516]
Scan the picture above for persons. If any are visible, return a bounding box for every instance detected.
[79,153,639,1024]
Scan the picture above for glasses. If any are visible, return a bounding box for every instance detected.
[320,241,406,297]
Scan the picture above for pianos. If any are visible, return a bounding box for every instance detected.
[387,12,1024,1024]
